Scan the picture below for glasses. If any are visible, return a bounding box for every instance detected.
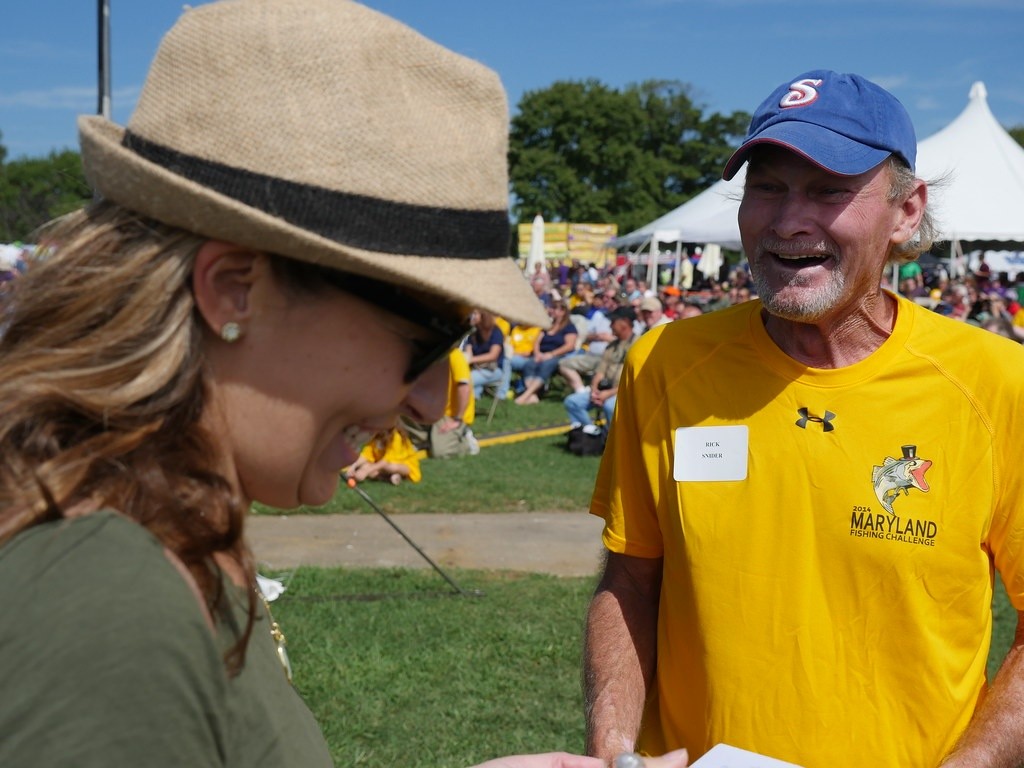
[265,250,480,383]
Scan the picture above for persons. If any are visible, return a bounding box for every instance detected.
[2,0,688,767]
[581,66,1024,764]
[332,232,1024,481]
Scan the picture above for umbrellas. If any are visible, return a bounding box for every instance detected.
[524,211,550,274]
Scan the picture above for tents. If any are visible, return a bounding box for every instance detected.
[601,79,1024,281]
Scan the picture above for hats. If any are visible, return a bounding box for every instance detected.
[75,0,559,335]
[604,305,637,320]
[721,70,918,178]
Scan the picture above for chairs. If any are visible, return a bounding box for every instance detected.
[480,343,515,429]
